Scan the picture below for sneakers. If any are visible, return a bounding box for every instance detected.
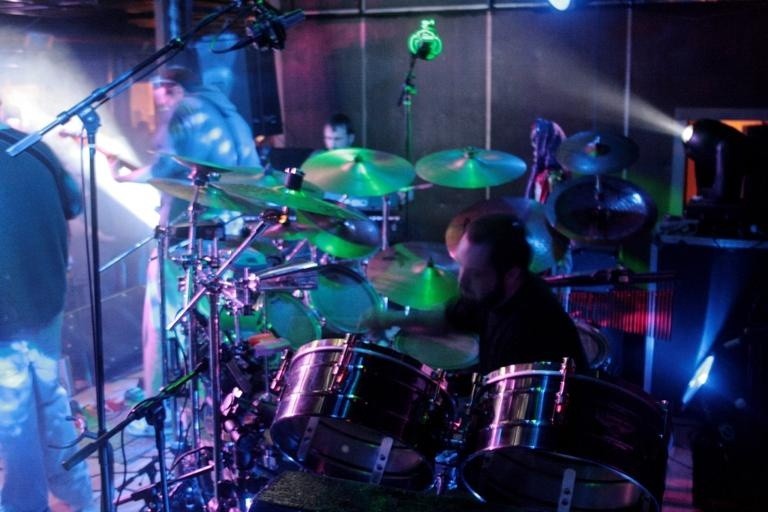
[126,411,173,436]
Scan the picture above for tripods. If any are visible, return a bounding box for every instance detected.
[118,395,192,492]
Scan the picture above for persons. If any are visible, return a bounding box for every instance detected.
[106,63,263,440]
[309,110,356,159]
[0,99,95,512]
[358,214,591,405]
[524,113,576,309]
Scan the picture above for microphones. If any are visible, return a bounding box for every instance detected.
[231,8,307,49]
[192,308,209,336]
[416,43,432,59]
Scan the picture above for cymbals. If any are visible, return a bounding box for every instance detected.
[416,147,528,188]
[443,195,569,278]
[544,174,659,245]
[300,148,416,195]
[296,200,382,261]
[371,240,470,312]
[167,151,261,180]
[150,176,260,217]
[555,129,639,174]
[207,180,362,222]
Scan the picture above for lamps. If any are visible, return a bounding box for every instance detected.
[680,123,704,151]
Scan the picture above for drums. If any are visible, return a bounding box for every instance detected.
[265,339,452,492]
[223,240,284,271]
[300,263,385,337]
[392,327,483,399]
[218,296,322,374]
[458,359,671,512]
